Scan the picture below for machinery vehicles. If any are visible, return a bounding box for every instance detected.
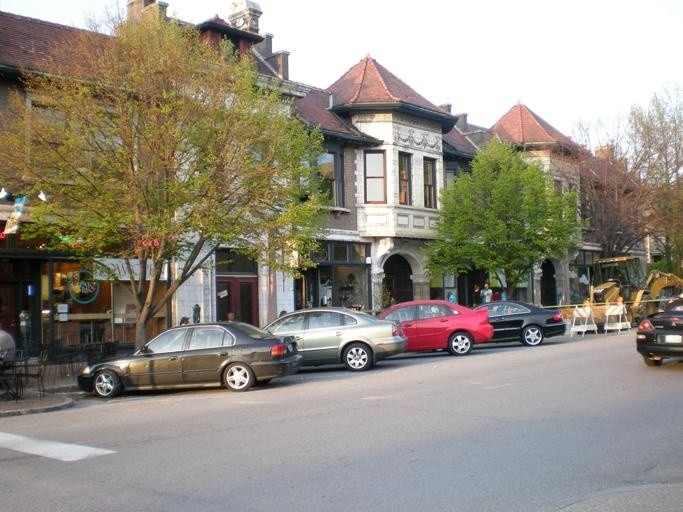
[559,254,683,329]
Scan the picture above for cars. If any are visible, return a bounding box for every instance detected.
[637,296,683,366]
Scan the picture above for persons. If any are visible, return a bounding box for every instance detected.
[226,311,236,322]
[180,316,191,327]
[446,290,457,304]
[0,328,17,389]
[479,283,493,303]
[472,283,482,307]
[491,288,500,301]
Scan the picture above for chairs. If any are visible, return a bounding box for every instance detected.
[0,338,119,403]
